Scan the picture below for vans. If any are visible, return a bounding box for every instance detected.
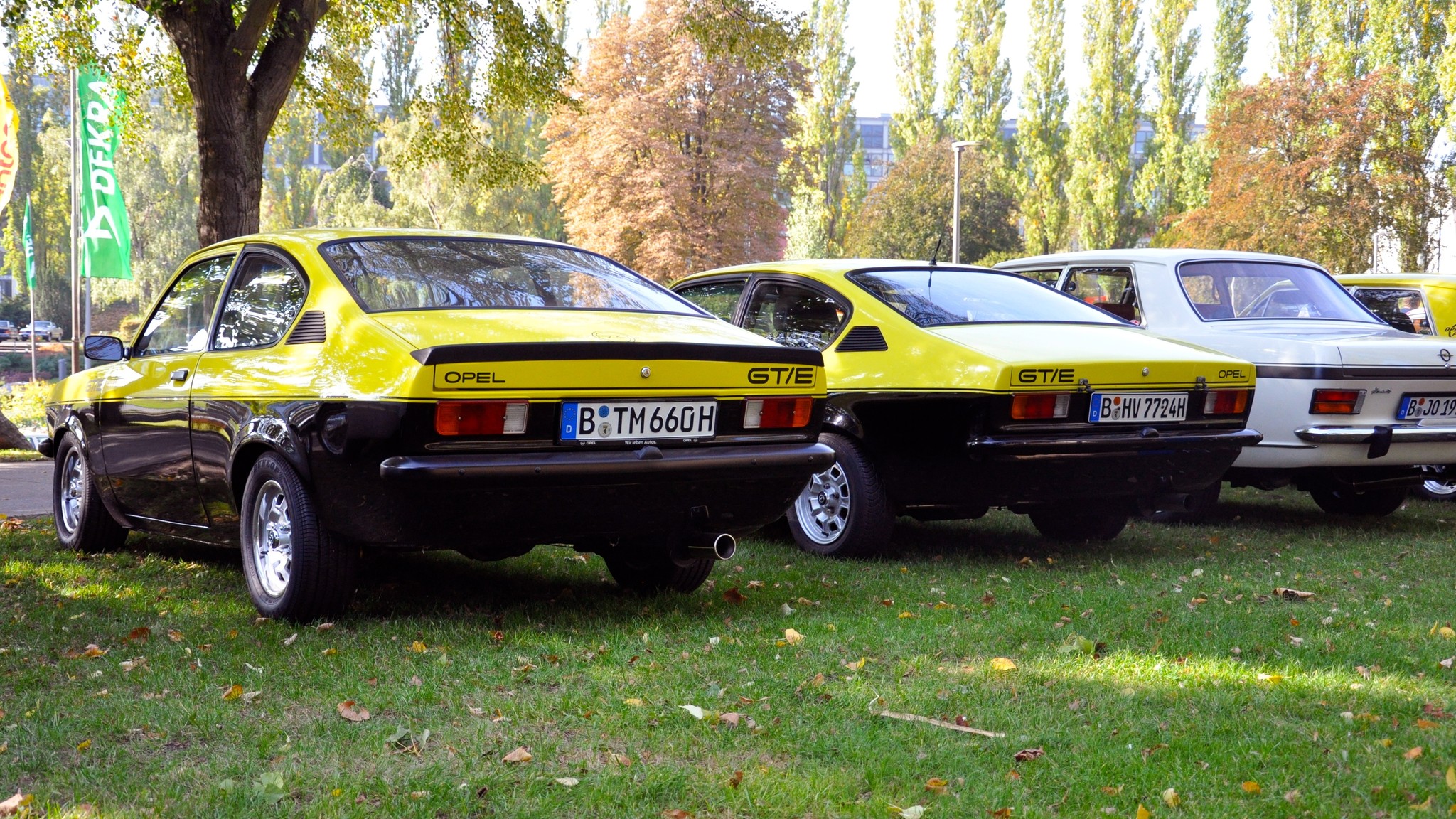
[1236,272,1455,340]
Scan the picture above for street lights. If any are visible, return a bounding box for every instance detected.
[951,140,980,264]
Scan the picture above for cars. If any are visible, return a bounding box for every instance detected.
[989,246,1456,519]
[19,320,65,342]
[665,257,1265,547]
[22,433,50,451]
[0,320,20,341]
[38,227,837,627]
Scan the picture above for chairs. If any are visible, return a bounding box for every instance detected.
[773,295,840,342]
